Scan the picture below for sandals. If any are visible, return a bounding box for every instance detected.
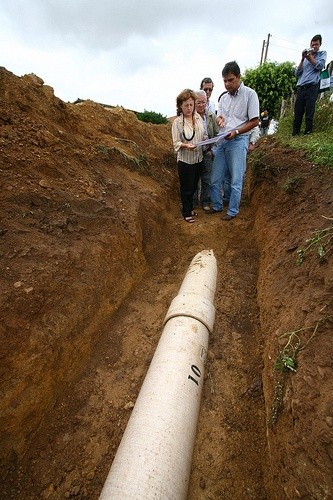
[192,210,198,216]
[184,215,196,223]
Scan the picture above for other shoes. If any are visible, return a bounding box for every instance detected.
[206,207,223,215]
[203,205,211,211]
[220,214,237,221]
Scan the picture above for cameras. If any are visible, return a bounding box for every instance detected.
[303,48,314,58]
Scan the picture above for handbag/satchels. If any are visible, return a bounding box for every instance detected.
[320,69,331,92]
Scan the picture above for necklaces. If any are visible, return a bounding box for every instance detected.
[183,113,195,141]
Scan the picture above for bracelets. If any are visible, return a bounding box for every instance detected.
[235,129,239,135]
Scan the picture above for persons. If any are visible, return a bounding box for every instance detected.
[260,110,271,135]
[193,91,220,213]
[218,91,261,206]
[171,89,212,223]
[205,61,260,220]
[200,78,215,113]
[291,35,327,136]
